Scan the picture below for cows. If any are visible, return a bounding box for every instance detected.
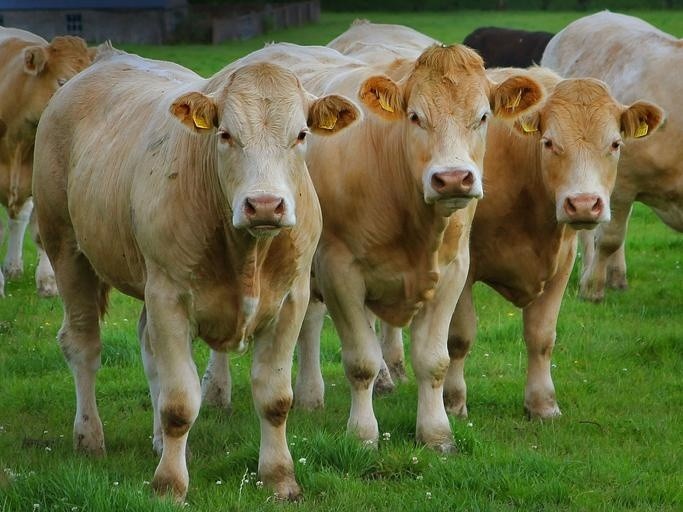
[214,41,545,456]
[30,39,367,503]
[324,15,671,422]
[541,7,683,302]
[461,25,556,69]
[0,27,100,297]
